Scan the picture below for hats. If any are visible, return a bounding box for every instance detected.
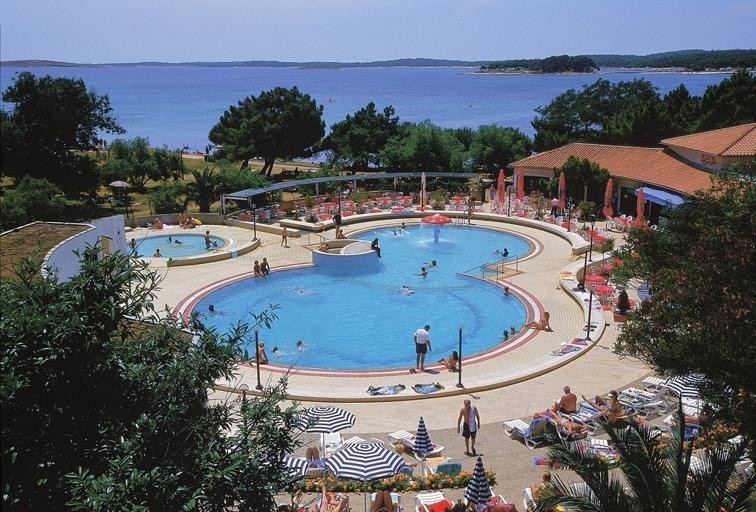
[563,385,570,390]
[608,394,614,397]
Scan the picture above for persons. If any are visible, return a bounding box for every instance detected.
[503,286,509,295]
[414,325,432,370]
[495,248,510,256]
[534,408,587,438]
[551,386,577,415]
[401,222,407,230]
[503,312,550,345]
[403,260,438,294]
[312,486,342,512]
[683,411,708,425]
[271,347,279,356]
[439,351,459,372]
[281,227,288,247]
[319,244,330,253]
[242,190,559,222]
[371,237,382,257]
[129,210,218,257]
[582,389,621,418]
[253,341,268,364]
[530,471,559,512]
[254,258,269,277]
[208,305,216,314]
[296,340,302,350]
[333,211,347,240]
[456,400,480,455]
[371,490,397,512]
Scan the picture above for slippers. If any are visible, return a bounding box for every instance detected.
[464,448,476,454]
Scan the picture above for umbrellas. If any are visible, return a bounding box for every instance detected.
[324,441,405,512]
[260,456,311,490]
[291,407,356,460]
[412,416,433,477]
[463,454,492,505]
